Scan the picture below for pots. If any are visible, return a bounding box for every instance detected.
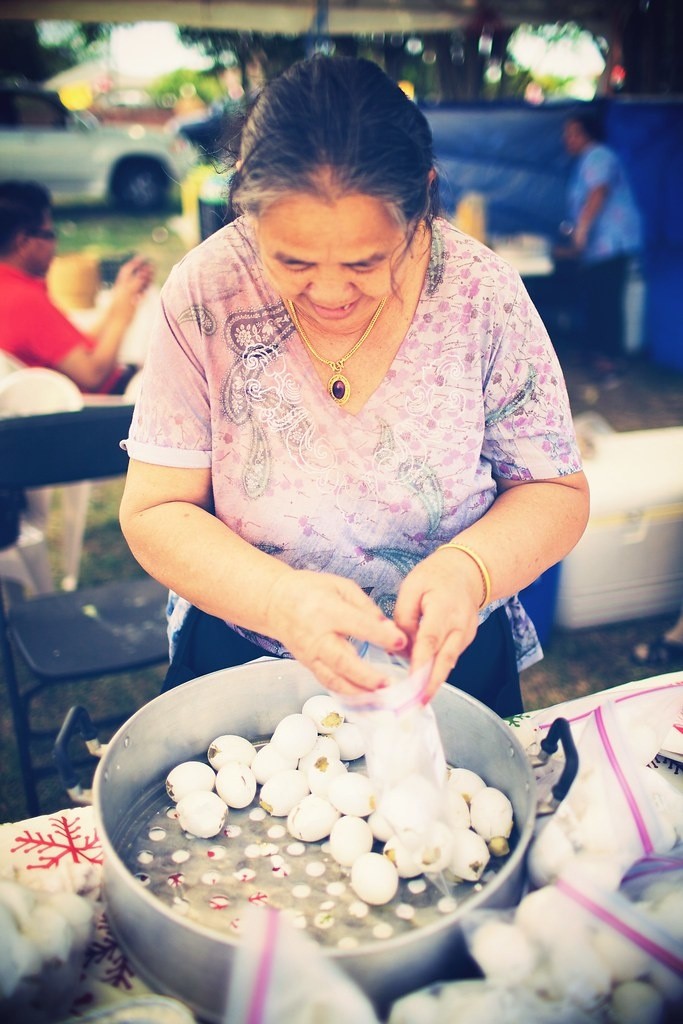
[49,655,581,1022]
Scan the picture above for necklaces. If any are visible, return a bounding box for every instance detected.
[289,298,387,405]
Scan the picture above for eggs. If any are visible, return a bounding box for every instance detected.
[166,694,515,907]
[390,773,683,1024]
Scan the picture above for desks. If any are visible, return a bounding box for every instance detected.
[0,669,683,1024]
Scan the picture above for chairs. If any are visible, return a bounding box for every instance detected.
[0,404,171,813]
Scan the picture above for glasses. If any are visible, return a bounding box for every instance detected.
[25,229,57,242]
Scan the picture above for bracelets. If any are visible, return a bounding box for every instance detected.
[437,544,490,611]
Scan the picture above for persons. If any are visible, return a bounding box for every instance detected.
[552,116,642,393]
[118,56,590,720]
[0,181,151,396]
